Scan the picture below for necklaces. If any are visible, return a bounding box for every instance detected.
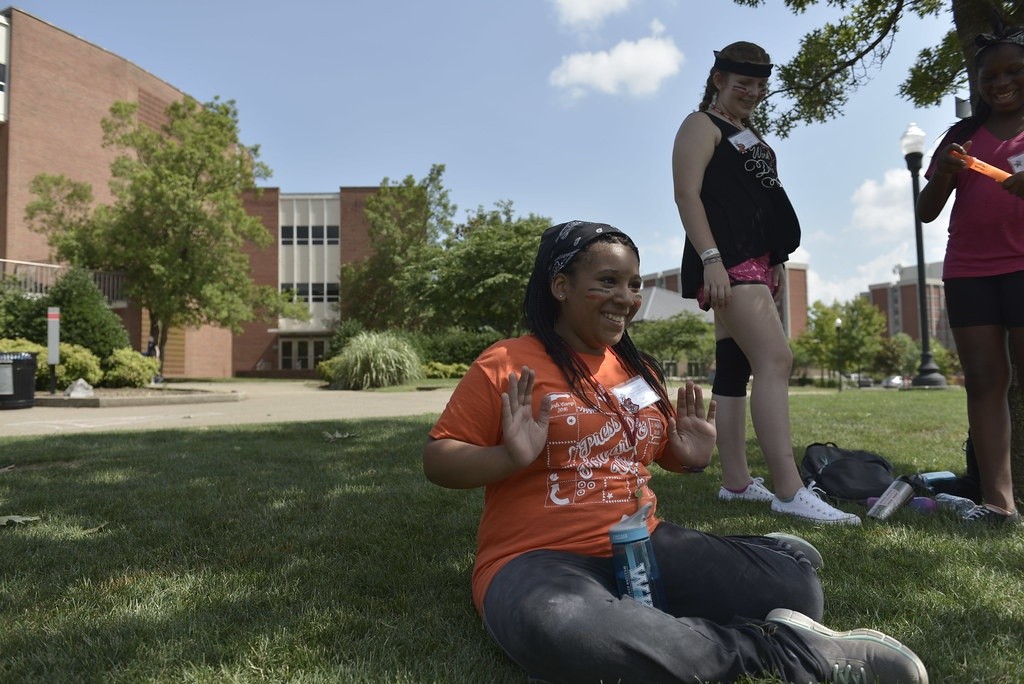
[561,338,645,498]
[672,40,863,528]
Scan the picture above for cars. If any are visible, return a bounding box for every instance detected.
[859,377,876,387]
[882,375,912,388]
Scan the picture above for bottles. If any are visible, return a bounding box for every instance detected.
[868,497,935,511]
[935,493,979,513]
[609,501,668,613]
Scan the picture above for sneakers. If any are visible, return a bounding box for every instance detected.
[719,477,774,502]
[771,480,861,525]
[961,501,1018,521]
[763,532,824,568]
[765,608,929,684]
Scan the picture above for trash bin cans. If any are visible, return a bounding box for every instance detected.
[0,352,40,410]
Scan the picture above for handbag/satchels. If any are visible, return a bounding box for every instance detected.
[801,441,895,500]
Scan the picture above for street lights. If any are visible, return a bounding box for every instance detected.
[900,121,950,387]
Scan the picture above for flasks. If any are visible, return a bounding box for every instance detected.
[866,475,917,519]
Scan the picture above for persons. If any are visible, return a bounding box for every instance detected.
[422,220,931,684]
[147,337,165,383]
[917,32,1024,522]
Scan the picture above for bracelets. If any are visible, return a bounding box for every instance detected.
[703,256,722,264]
[700,247,719,261]
